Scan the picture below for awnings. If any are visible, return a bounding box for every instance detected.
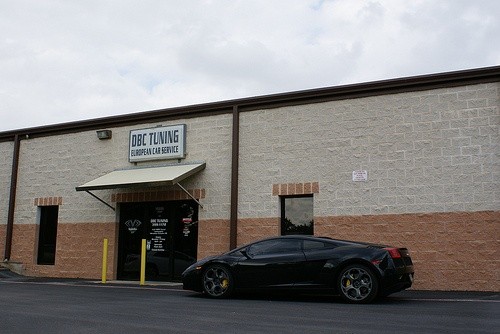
[74,162,207,212]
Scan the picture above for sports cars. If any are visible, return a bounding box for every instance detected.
[182,234,415,304]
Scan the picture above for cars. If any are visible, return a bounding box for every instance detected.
[127,248,197,278]
